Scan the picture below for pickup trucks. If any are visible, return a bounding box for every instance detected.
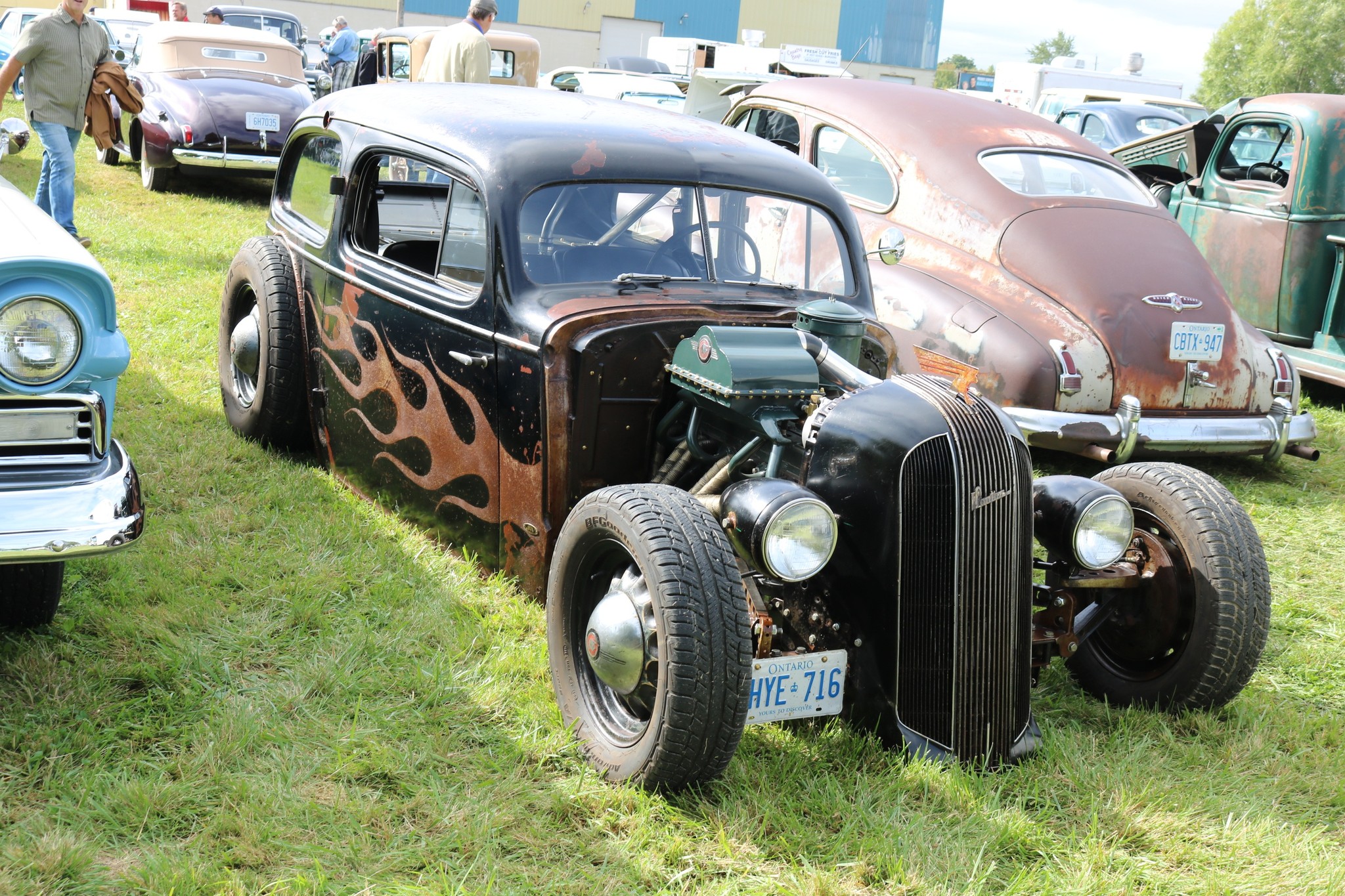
[1169,92,1344,388]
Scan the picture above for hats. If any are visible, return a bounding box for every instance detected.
[203,7,223,16]
[470,0,498,15]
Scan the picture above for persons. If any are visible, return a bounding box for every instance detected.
[0,0,115,248]
[962,76,977,90]
[755,110,800,153]
[352,28,387,87]
[202,7,232,26]
[1250,125,1271,139]
[171,1,191,22]
[389,0,498,184]
[319,16,360,92]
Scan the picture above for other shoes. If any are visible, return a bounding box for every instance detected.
[71,233,91,249]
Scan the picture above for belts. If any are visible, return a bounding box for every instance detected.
[333,61,343,66]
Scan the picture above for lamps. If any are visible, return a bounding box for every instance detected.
[583,1,591,13]
[679,12,688,24]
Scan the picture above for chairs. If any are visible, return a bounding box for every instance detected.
[378,240,485,276]
[552,245,690,282]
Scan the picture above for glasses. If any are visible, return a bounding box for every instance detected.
[492,15,496,21]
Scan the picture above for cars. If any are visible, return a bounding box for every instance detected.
[0,118,144,639]
[634,76,1322,466]
[0,6,1305,193]
[217,83,1271,800]
